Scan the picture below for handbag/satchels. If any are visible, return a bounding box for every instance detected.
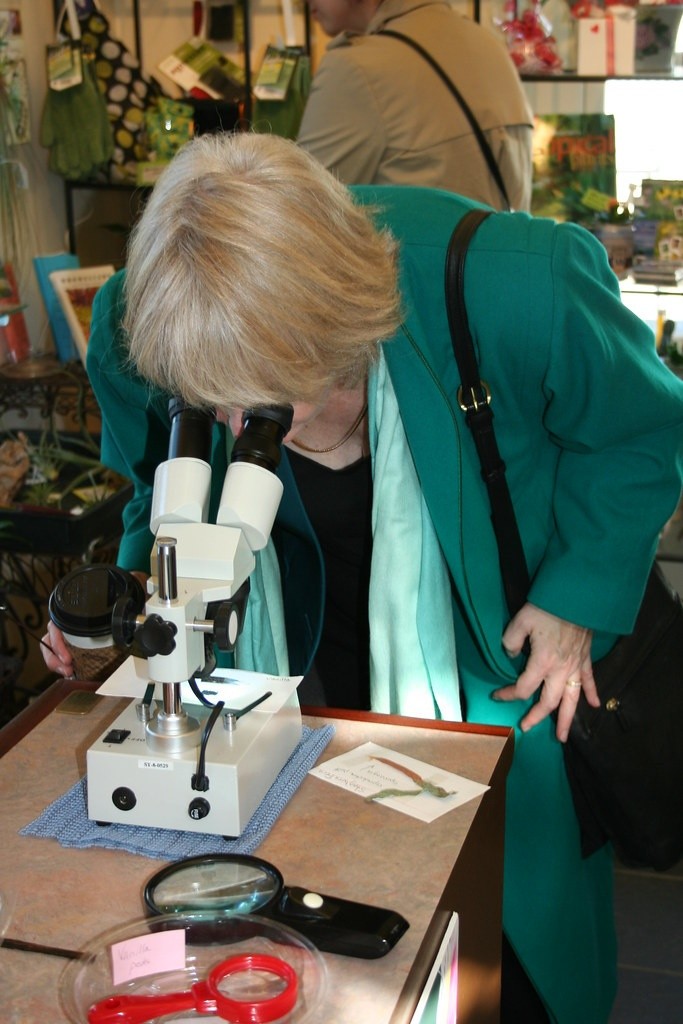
[553,561,682,872]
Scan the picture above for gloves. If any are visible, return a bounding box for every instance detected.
[38,42,113,183]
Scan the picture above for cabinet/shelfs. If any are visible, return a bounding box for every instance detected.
[520,74,683,296]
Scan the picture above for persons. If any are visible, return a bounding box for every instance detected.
[296,0,534,217]
[38,135,683,1024]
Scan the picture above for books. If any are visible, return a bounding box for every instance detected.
[35,252,117,371]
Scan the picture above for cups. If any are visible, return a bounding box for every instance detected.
[599,226,635,280]
[635,4,683,72]
[49,566,145,682]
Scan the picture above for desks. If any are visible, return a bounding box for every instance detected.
[0,678,517,1024]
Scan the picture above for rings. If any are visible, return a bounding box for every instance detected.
[566,680,581,686]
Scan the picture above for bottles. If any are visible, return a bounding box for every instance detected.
[656,310,666,345]
[525,0,552,74]
[496,0,526,74]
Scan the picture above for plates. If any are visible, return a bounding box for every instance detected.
[57,912,329,1023]
